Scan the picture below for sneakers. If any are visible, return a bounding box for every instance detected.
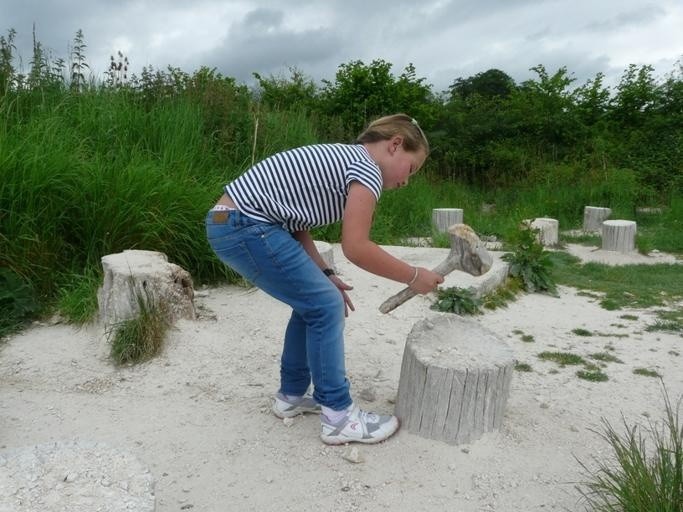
[270,384,321,419]
[321,407,400,446]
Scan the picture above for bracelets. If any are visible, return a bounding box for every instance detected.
[322,268,336,278]
[406,266,417,285]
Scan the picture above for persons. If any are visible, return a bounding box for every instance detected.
[204,112,446,444]
